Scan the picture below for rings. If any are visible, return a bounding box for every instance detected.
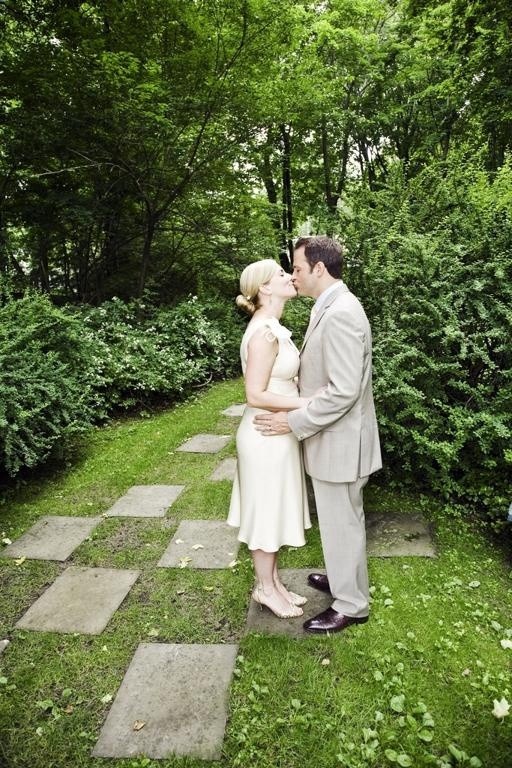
[269,425,272,432]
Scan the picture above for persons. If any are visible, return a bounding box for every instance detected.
[251,236,384,633]
[226,258,327,621]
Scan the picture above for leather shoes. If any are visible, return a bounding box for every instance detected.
[307,573,332,593]
[303,606,369,634]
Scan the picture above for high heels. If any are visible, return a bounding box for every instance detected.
[251,578,308,619]
